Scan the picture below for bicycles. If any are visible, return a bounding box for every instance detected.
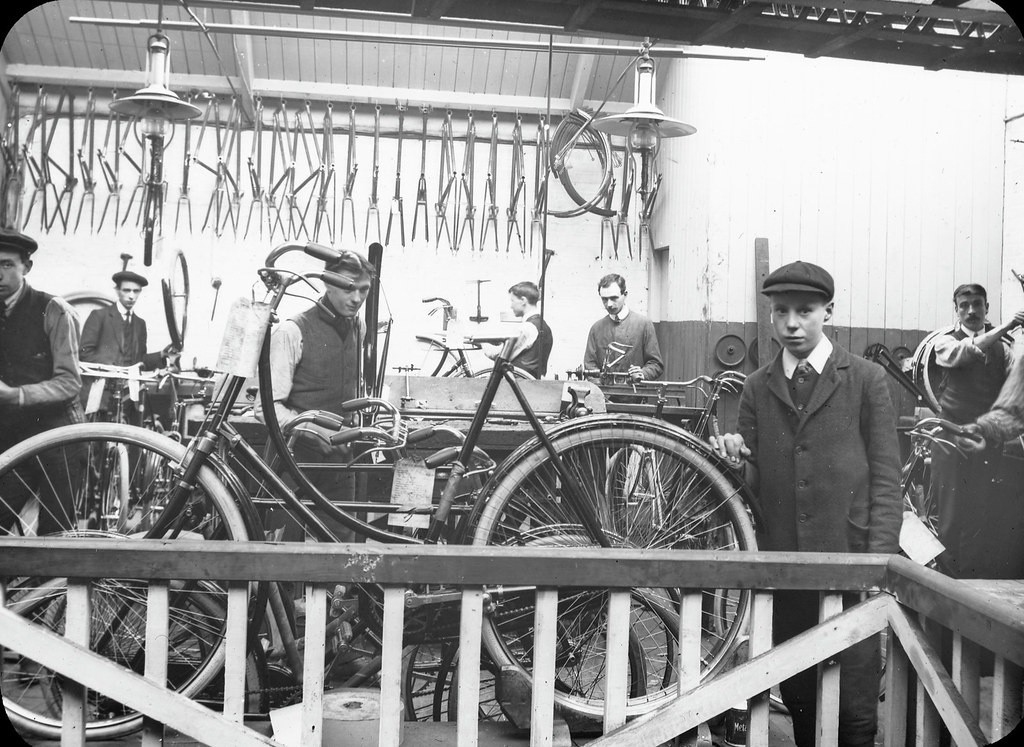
[0,241,1024,740]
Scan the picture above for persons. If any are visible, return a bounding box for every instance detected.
[0,227,89,688]
[933,282,1024,579]
[252,249,375,640]
[707,260,905,747]
[80,270,179,424]
[583,273,664,536]
[508,280,555,381]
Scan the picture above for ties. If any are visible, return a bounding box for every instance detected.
[0,301,7,329]
[615,315,620,323]
[794,359,812,376]
[124,311,131,324]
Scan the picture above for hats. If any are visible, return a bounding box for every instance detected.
[113,271,148,287]
[761,258,834,303]
[0,228,38,254]
